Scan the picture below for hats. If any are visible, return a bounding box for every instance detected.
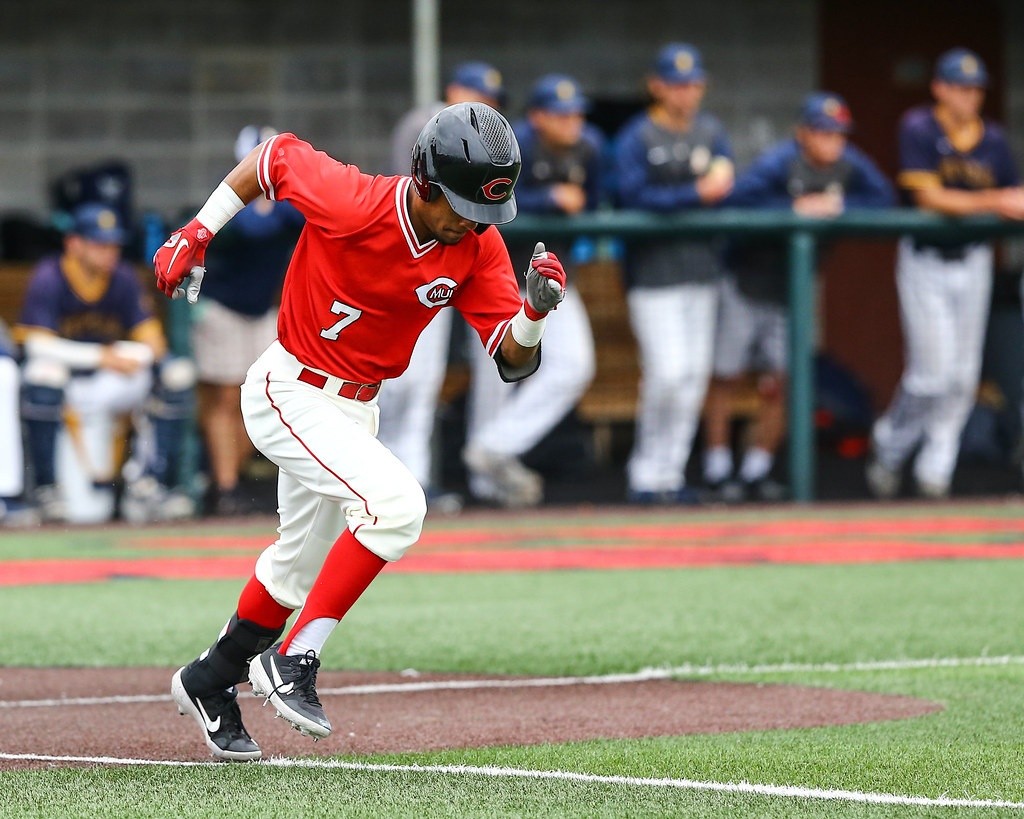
[452,61,506,100]
[76,206,124,243]
[650,41,707,84]
[935,51,986,89]
[797,94,852,136]
[528,74,589,112]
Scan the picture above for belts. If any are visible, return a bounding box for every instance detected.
[297,367,383,402]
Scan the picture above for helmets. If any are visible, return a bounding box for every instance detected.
[410,101,523,225]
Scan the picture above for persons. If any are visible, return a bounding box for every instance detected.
[188,121,307,515]
[606,40,739,506]
[152,101,566,762]
[701,89,897,506]
[374,58,514,492]
[0,324,45,523]
[866,52,1023,501]
[458,72,613,509]
[16,203,193,525]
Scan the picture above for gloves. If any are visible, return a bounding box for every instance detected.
[152,216,215,305]
[524,241,567,323]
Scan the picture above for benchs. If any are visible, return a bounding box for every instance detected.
[0,247,790,467]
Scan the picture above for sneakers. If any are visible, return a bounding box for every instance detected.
[247,642,331,744]
[170,648,263,760]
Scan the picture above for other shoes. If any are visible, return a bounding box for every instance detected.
[918,473,949,498]
[0,495,39,531]
[33,484,62,521]
[463,442,544,504]
[628,486,697,503]
[732,473,787,501]
[868,432,902,498]
[217,485,255,513]
[695,470,747,503]
